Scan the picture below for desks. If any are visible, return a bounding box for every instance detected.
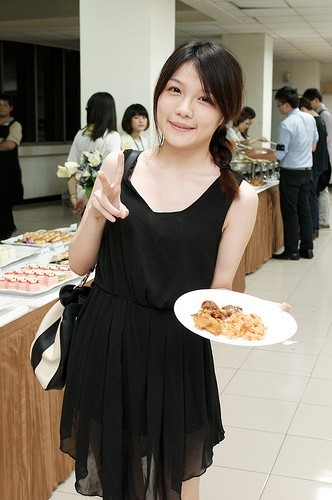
[0,178,284,500]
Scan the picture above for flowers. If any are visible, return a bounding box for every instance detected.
[56,150,104,199]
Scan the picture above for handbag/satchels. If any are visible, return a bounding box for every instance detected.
[29,285,89,392]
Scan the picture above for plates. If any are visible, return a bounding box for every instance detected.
[1,229,74,249]
[0,261,80,297]
[0,244,49,268]
[48,251,69,267]
[174,289,297,347]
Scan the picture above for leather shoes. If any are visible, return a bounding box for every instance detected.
[297,249,314,259]
[271,251,300,261]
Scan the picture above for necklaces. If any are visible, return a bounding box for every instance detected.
[130,133,145,150]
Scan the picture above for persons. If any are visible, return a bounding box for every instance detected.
[241,107,256,140]
[297,97,327,240]
[60,40,292,500]
[303,88,332,228]
[247,85,319,259]
[122,103,150,152]
[0,97,24,240]
[65,92,121,220]
[227,113,269,179]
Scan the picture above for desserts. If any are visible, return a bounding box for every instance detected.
[0,264,74,292]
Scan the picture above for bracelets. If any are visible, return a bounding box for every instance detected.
[69,192,77,198]
